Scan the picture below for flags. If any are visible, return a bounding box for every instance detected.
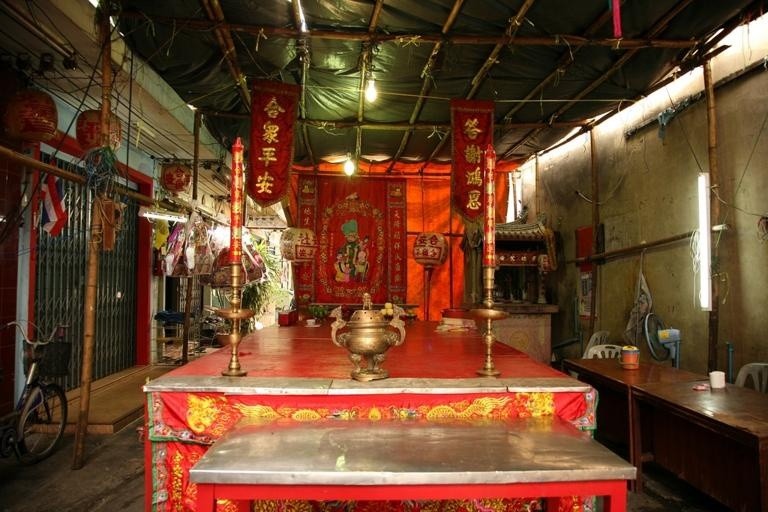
[37,151,67,237]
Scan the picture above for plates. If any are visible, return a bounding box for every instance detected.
[305,324,321,328]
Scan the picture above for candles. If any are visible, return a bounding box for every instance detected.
[483,143,497,268]
[228,137,248,264]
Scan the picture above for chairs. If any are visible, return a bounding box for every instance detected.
[735,357,767,394]
[582,329,609,361]
[589,344,623,358]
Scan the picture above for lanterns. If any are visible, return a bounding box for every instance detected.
[0,87,58,142]
[160,164,191,195]
[412,231,448,270]
[75,108,121,153]
[278,225,318,266]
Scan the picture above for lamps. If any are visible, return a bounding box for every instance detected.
[361,42,377,102]
[341,124,356,176]
[695,171,715,313]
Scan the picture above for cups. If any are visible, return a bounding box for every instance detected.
[707,371,726,389]
[306,320,315,325]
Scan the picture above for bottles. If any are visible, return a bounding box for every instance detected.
[620,345,641,370]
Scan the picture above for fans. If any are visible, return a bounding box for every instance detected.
[640,311,681,368]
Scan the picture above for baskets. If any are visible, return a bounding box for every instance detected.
[22,340,71,376]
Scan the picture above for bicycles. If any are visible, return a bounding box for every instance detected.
[1,320,71,467]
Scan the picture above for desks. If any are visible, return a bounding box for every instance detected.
[562,351,766,512]
[183,416,638,512]
[143,313,598,512]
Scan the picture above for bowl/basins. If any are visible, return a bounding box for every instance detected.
[440,307,466,318]
